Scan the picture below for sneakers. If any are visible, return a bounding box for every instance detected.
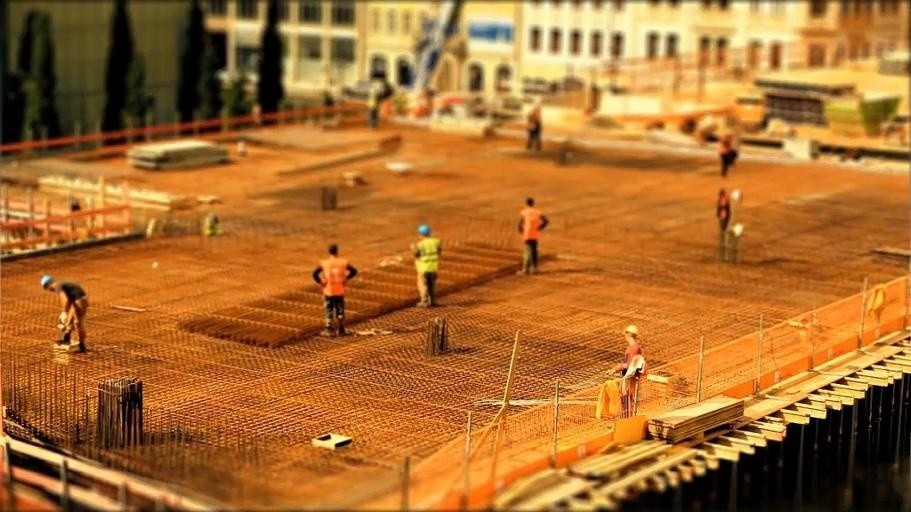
[416,302,428,308]
[72,346,85,354]
[321,327,336,337]
[339,329,345,336]
[56,339,70,345]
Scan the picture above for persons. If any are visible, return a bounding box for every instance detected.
[363,70,390,129]
[40,274,90,353]
[715,189,734,262]
[517,197,550,276]
[408,223,443,308]
[606,325,643,416]
[312,243,359,339]
[718,130,733,178]
[523,105,544,152]
[414,87,437,120]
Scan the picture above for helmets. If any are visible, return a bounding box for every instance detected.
[623,326,638,336]
[418,224,430,235]
[41,275,51,287]
